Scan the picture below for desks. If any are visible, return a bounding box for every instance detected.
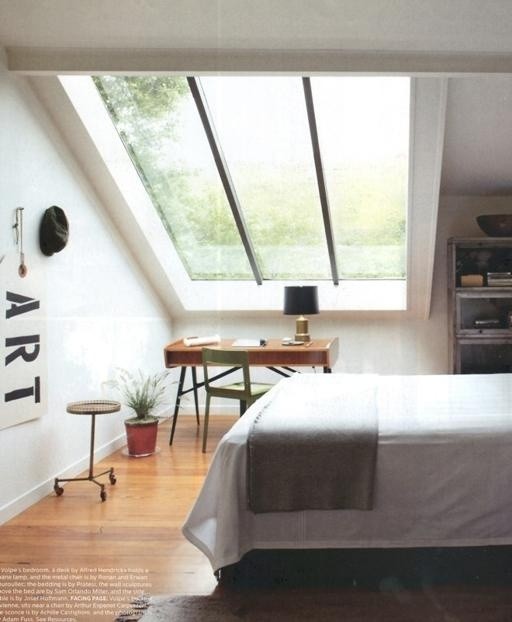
[158,332,343,451]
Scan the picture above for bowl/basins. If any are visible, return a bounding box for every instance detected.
[476,213,510,237]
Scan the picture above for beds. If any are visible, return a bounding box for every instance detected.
[181,369,511,593]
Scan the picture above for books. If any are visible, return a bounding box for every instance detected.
[456,269,511,330]
[185,336,221,348]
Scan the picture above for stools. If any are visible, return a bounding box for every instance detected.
[52,400,117,501]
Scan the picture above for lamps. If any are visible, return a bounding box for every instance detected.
[282,284,321,346]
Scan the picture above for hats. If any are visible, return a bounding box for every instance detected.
[39,205,70,256]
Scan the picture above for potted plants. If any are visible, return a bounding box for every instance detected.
[112,366,184,457]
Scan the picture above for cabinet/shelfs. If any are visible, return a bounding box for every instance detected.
[445,233,512,374]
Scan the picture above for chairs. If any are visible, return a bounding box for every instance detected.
[197,346,274,455]
